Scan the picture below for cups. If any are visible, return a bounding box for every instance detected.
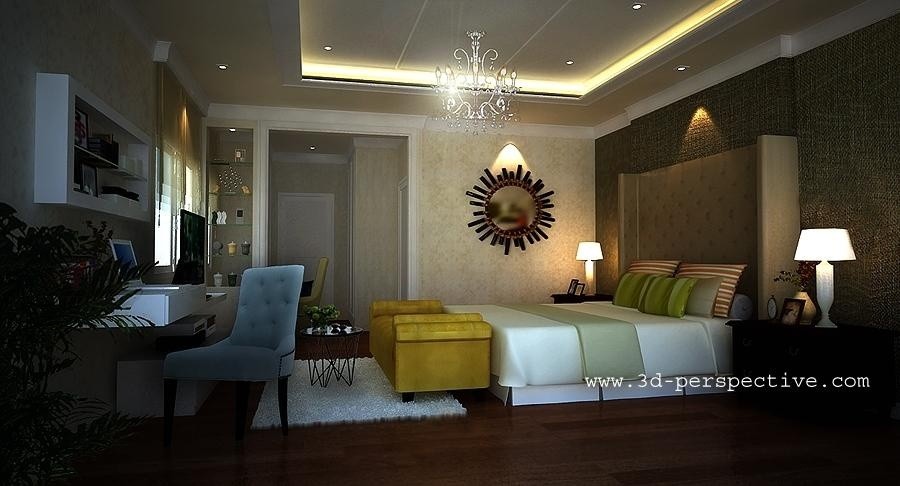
[214,271,223,287]
[228,241,237,256]
[228,272,237,287]
[241,242,249,255]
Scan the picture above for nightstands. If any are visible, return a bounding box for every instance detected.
[551,293,614,304]
[732,320,892,422]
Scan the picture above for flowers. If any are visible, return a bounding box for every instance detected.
[774,260,822,293]
[303,304,339,323]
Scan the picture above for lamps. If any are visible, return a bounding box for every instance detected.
[793,228,856,328]
[431,27,520,133]
[576,241,603,296]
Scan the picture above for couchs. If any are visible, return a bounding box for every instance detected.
[369,301,492,402]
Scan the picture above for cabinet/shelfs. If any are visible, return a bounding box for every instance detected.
[203,116,259,291]
[34,72,152,221]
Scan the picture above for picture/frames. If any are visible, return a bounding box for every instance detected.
[780,298,806,326]
[110,238,143,287]
[567,279,578,294]
[574,282,584,295]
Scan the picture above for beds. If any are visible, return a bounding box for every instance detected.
[444,261,751,406]
[299,327,363,389]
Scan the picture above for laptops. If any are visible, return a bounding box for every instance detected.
[110,237,180,290]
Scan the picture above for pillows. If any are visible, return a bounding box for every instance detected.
[638,276,697,318]
[615,273,650,309]
[684,276,720,319]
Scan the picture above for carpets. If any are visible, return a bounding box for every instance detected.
[234,358,251,373]
[240,358,469,429]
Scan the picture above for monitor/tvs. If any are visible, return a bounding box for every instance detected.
[179,209,206,285]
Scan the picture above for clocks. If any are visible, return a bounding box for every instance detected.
[767,296,778,320]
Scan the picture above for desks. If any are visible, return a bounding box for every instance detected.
[43,272,204,331]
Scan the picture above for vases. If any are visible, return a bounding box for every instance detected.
[313,321,328,334]
[793,292,816,325]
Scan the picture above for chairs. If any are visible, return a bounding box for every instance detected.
[295,258,330,318]
[163,265,304,448]
